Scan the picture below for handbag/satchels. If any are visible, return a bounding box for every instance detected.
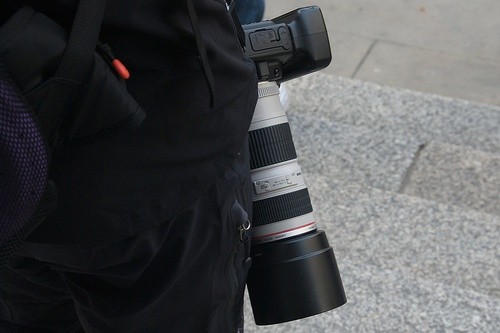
[0,57,76,274]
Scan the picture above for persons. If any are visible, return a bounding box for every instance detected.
[0,0,259,332]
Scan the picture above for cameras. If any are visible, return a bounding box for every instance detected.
[244,5,348,327]
